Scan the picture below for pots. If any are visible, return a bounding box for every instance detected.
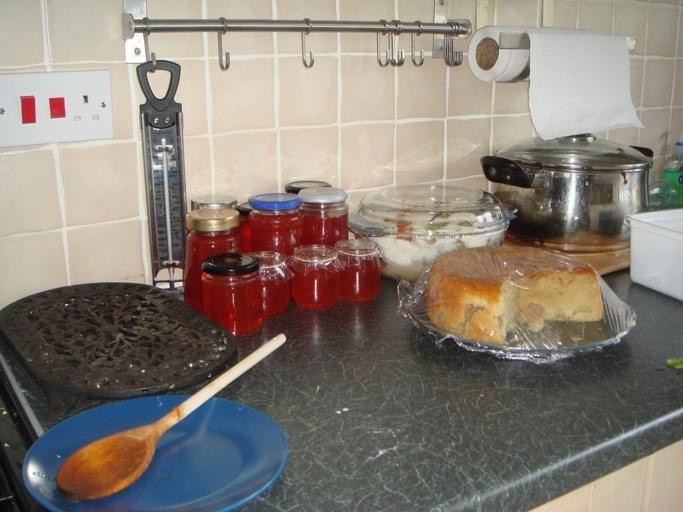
[481,133,654,252]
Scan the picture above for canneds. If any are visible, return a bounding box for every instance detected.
[183,181,382,336]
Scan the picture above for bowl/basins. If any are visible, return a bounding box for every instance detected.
[349,183,516,285]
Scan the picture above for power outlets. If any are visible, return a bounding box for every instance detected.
[0,67,116,149]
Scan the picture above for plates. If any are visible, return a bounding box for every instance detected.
[396,288,637,358]
[23,392,285,510]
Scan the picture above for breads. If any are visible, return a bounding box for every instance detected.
[422,242,607,347]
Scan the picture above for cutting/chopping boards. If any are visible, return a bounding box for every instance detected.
[501,238,629,277]
